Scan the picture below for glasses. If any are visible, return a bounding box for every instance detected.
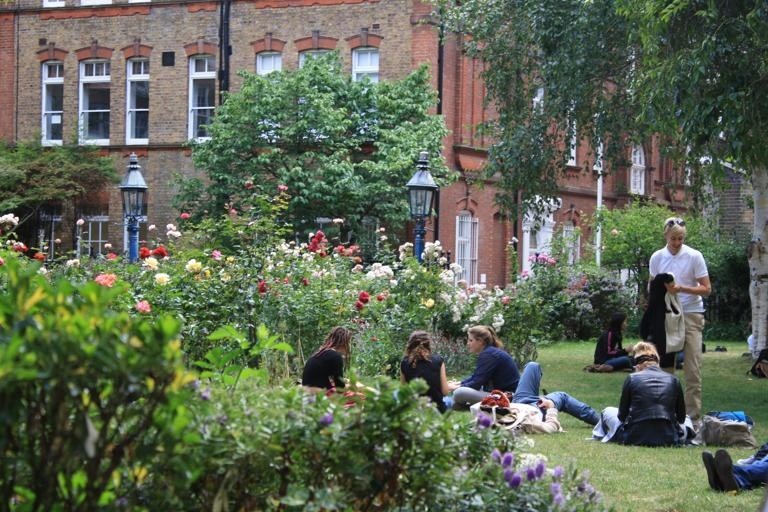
[664,220,686,230]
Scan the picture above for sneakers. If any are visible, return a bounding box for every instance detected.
[702,449,740,496]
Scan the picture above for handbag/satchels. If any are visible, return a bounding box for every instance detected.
[665,290,685,353]
[695,417,757,450]
[703,411,752,427]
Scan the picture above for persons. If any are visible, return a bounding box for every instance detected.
[400,332,451,420]
[649,218,711,444]
[593,314,639,369]
[703,444,764,491]
[301,328,364,405]
[457,328,521,412]
[603,342,687,444]
[474,360,601,435]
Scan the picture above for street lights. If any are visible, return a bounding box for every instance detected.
[401,149,439,258]
[404,150,439,269]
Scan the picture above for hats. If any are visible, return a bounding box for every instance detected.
[481,390,510,408]
